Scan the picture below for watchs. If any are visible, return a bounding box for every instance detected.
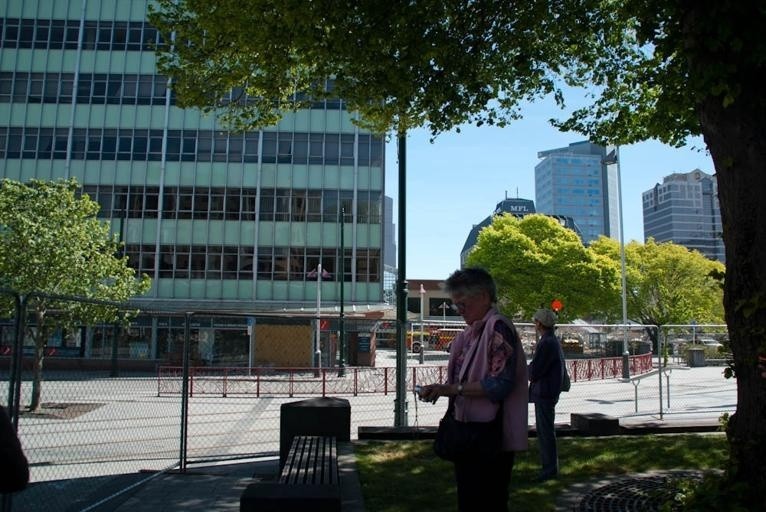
[457,384,464,396]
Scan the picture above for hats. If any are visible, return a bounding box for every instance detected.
[533,309,557,327]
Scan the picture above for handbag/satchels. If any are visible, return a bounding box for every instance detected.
[432,407,505,470]
[561,367,570,392]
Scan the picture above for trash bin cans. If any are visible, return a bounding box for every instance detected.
[687,348,705,367]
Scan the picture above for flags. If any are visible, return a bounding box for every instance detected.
[600,150,618,166]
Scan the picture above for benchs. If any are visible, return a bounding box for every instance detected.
[279,432,340,491]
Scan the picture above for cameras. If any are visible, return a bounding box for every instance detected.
[415,385,432,402]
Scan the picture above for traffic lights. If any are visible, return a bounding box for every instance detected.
[552,299,564,313]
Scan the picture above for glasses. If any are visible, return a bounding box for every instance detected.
[450,301,467,312]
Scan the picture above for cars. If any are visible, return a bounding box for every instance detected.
[373,321,584,356]
[648,333,725,353]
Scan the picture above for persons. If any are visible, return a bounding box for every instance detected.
[527,308,571,483]
[418,268,530,509]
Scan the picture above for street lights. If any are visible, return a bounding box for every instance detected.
[308,264,332,377]
[599,148,630,381]
[417,283,428,364]
[439,302,449,320]
